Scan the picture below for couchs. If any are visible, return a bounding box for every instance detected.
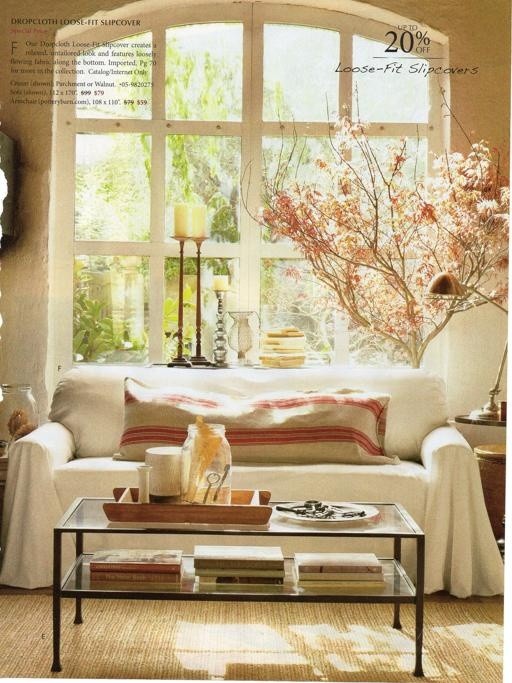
[0,365,504,598]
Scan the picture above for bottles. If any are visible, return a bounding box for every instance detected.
[178,423,232,505]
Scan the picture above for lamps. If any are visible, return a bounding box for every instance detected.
[428,271,508,421]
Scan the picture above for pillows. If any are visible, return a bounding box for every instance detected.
[112,376,400,465]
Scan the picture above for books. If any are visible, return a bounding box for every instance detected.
[90,544,388,588]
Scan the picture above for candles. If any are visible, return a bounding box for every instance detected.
[212,274,228,292]
[192,206,205,236]
[173,203,188,235]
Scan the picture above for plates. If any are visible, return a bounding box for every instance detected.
[271,502,381,525]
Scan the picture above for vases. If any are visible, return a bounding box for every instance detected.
[228,310,256,362]
